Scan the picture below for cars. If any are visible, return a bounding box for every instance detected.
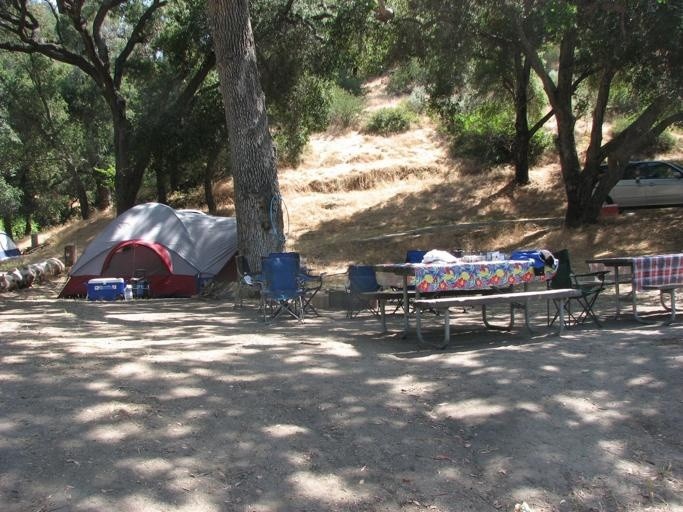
[581,160,682,210]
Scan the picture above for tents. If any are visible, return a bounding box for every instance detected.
[0,231,21,262]
[57,202,237,299]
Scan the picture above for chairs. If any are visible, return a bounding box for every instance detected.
[545,250,609,327]
[344,263,381,318]
[231,252,327,325]
[385,252,439,316]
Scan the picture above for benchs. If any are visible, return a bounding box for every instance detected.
[584,278,632,286]
[414,288,581,348]
[642,283,682,327]
[358,289,513,339]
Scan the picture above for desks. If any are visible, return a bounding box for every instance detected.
[372,259,559,348]
[583,254,682,327]
